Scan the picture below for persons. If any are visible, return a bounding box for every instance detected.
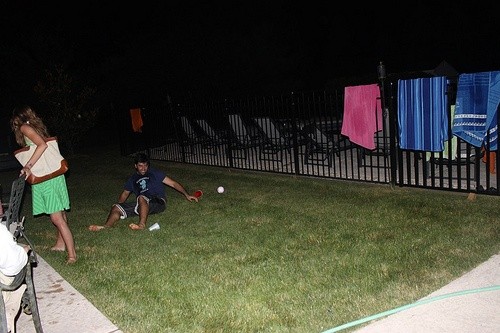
[88,153,198,231]
[11,104,77,264]
[0,200,31,315]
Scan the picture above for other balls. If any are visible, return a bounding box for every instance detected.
[217,186,224,193]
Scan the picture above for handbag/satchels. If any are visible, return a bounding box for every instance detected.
[13,135,68,184]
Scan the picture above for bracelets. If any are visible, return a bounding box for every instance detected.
[27,161,34,168]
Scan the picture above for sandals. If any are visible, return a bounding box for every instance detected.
[66,252,79,267]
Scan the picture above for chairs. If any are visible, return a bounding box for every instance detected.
[179,104,488,181]
[0,260,43,333]
[0,173,37,262]
